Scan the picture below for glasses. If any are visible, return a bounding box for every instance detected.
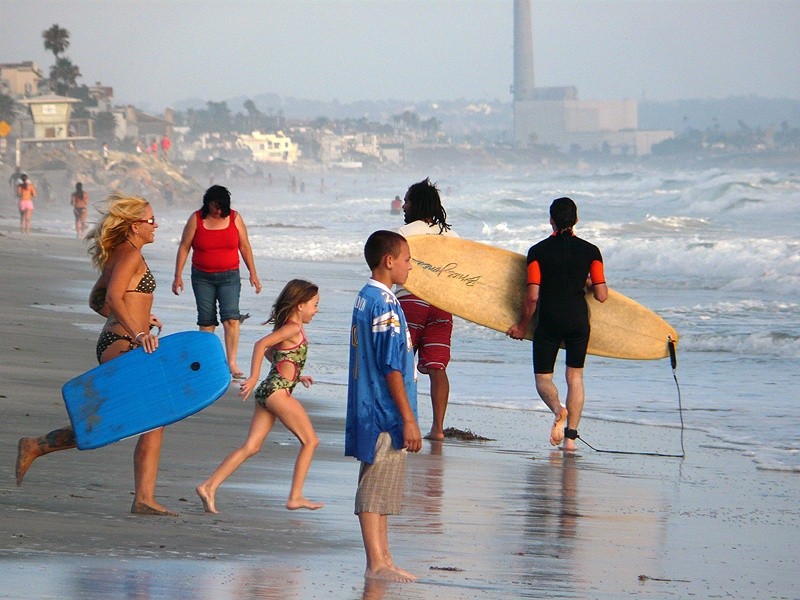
[132,217,155,226]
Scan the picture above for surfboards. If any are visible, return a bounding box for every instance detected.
[62,331,231,450]
[400,234,679,360]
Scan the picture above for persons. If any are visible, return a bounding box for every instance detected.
[8,167,52,234]
[101,133,170,170]
[209,171,453,215]
[70,182,89,239]
[344,230,421,582]
[394,177,453,439]
[15,194,179,516]
[172,184,261,378]
[195,279,324,513]
[505,197,608,449]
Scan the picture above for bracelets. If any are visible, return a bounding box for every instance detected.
[134,332,146,340]
